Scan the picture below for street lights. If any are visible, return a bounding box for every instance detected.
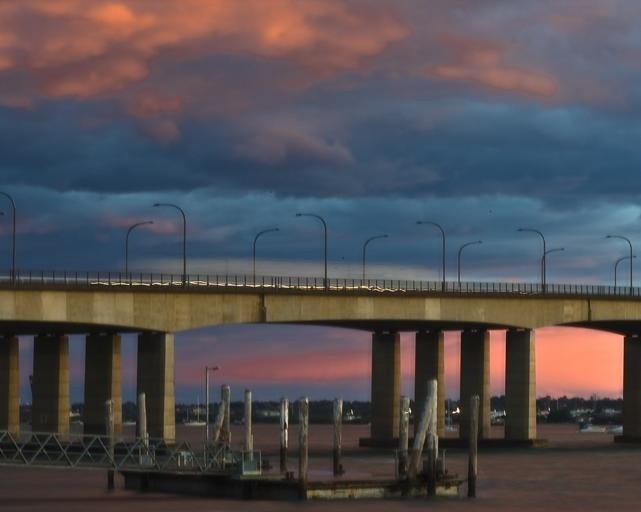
[206,366,219,465]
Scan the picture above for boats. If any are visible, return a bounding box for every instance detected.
[609,426,623,434]
[576,425,606,433]
